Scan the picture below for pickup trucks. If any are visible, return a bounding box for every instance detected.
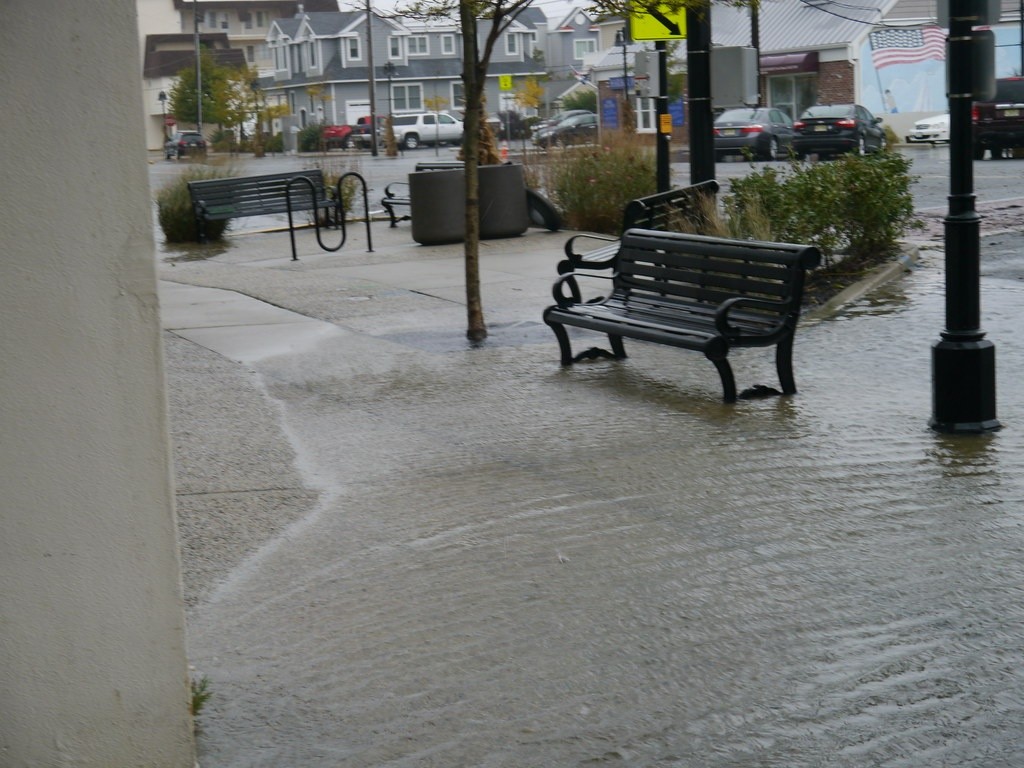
[351,125,385,148]
[324,115,388,148]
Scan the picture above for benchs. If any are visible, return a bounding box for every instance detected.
[381,161,471,228]
[557,178,720,307]
[542,227,823,403]
[187,168,341,245]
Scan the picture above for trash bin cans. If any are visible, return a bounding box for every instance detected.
[408,167,467,245]
[477,160,529,240]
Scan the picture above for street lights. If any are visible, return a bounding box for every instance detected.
[383,60,400,115]
[614,26,635,102]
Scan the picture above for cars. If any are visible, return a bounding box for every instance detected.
[710,106,797,160]
[790,101,890,161]
[910,113,952,148]
[530,114,600,149]
[530,108,594,136]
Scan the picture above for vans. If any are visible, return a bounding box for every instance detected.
[385,111,466,149]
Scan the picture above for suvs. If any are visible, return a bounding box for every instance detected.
[163,131,206,160]
[972,74,1024,163]
